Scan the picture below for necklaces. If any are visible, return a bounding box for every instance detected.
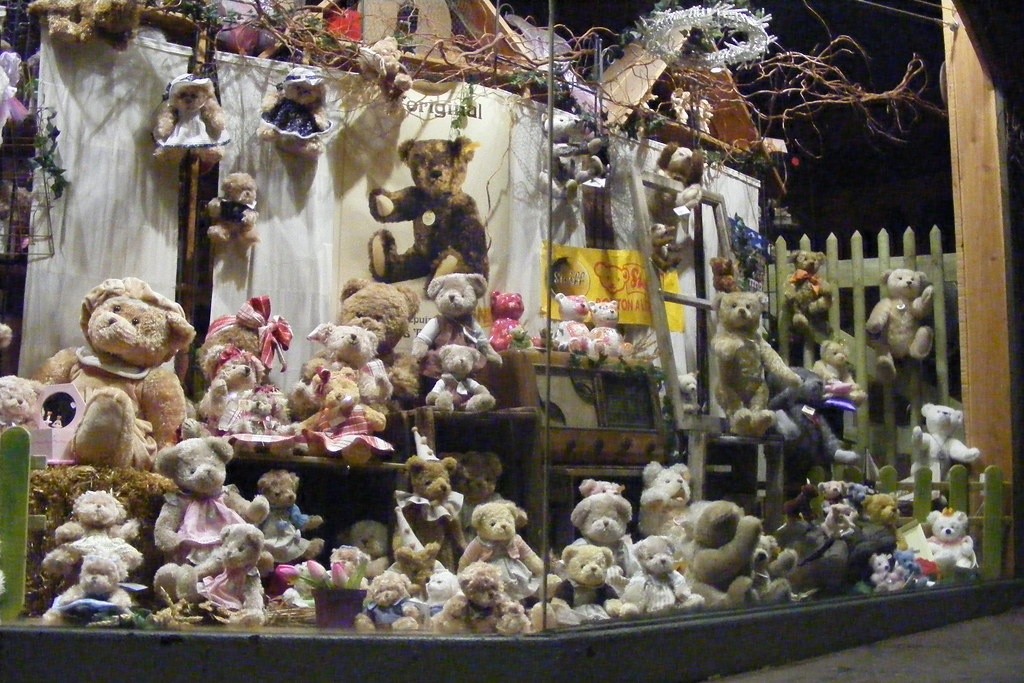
[932,434,949,459]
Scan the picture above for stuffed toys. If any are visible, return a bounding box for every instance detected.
[787,251,832,327]
[710,258,867,464]
[647,141,705,268]
[204,173,261,245]
[866,267,935,379]
[0,273,981,634]
[537,116,605,199]
[153,76,231,166]
[259,66,330,159]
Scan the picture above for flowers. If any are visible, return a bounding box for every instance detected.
[276,560,368,591]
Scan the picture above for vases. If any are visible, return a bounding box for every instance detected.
[311,589,367,631]
